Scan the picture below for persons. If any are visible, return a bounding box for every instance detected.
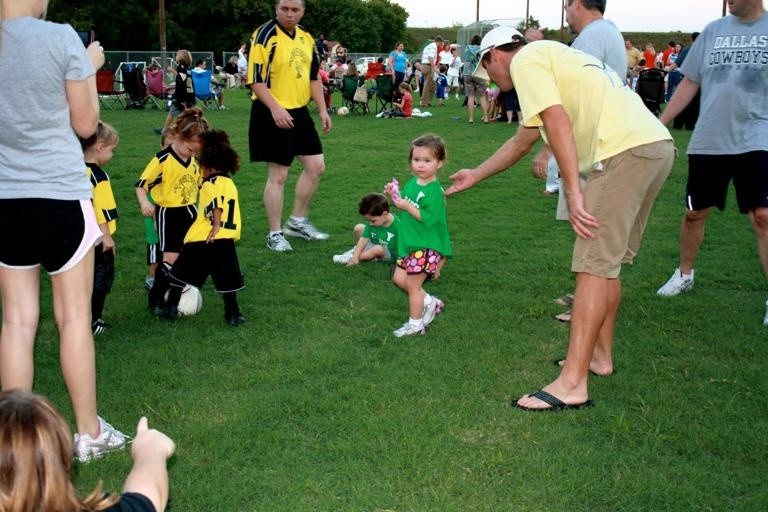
[238,43,248,79]
[327,57,348,78]
[321,39,329,55]
[153,49,195,135]
[316,32,329,64]
[443,47,462,101]
[463,35,489,123]
[1,1,135,464]
[485,81,522,124]
[436,64,447,105]
[191,59,232,112]
[376,57,386,73]
[667,43,682,102]
[662,41,676,67]
[77,121,119,337]
[383,134,453,338]
[120,63,147,110]
[163,129,246,327]
[333,192,401,267]
[328,40,343,64]
[670,32,701,131]
[375,82,413,119]
[145,63,176,100]
[531,1,628,323]
[388,41,407,88]
[438,43,452,73]
[656,0,768,324]
[643,42,656,68]
[318,58,335,114]
[143,124,176,291]
[624,39,642,91]
[444,25,677,411]
[135,107,211,316]
[419,36,444,107]
[1,388,177,512]
[220,56,240,89]
[403,59,424,94]
[245,0,332,252]
[342,62,369,112]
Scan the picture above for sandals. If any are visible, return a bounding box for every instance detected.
[469,119,473,124]
[482,118,488,123]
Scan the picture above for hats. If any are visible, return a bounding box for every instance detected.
[472,28,524,80]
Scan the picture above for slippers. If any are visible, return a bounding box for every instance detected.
[511,387,593,412]
[556,358,618,376]
[555,310,574,322]
[554,294,572,305]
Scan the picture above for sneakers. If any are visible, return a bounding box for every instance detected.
[423,296,444,327]
[333,255,352,265]
[149,297,163,317]
[657,269,694,296]
[227,311,244,327]
[165,304,176,319]
[763,301,768,327]
[376,111,384,118]
[73,418,126,463]
[266,232,292,251]
[282,220,329,241]
[203,105,210,112]
[146,278,155,291]
[343,246,356,255]
[394,319,424,338]
[91,318,112,337]
[154,127,166,135]
[218,106,229,111]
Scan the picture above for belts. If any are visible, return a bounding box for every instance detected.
[422,63,432,66]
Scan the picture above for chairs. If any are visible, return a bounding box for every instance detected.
[365,63,385,80]
[96,70,124,111]
[145,68,175,112]
[191,69,220,112]
[215,66,230,89]
[375,75,393,115]
[307,96,327,114]
[341,75,370,116]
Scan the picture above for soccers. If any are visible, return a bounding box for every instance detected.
[412,108,420,115]
[337,107,349,117]
[164,285,202,316]
